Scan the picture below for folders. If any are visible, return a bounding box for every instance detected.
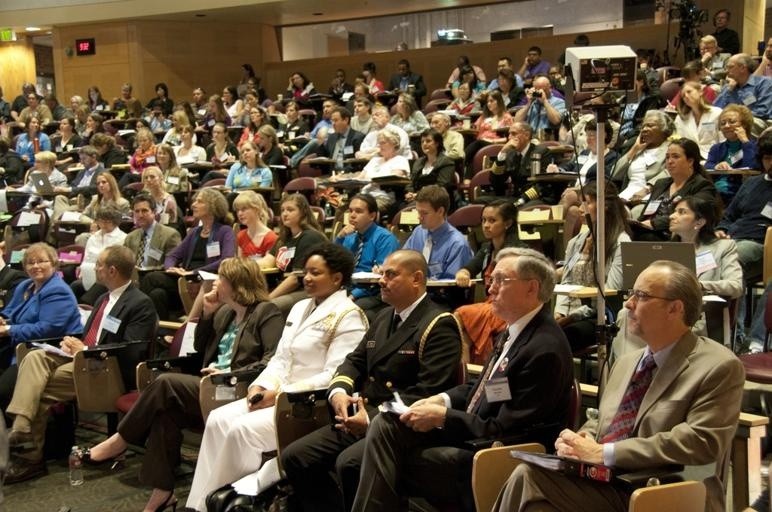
[510,449,613,483]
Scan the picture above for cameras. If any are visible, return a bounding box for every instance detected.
[532,90,541,97]
[669,0,709,40]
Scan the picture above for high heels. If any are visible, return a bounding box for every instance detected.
[73,445,130,472]
[154,490,178,512]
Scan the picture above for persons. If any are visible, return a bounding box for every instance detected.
[184,242,370,511]
[0,241,84,409]
[610,110,676,222]
[140,187,236,317]
[51,146,104,218]
[558,92,620,157]
[328,130,410,195]
[224,141,272,194]
[69,206,128,308]
[5,152,67,217]
[545,119,621,204]
[487,56,523,91]
[74,256,285,512]
[0,55,206,170]
[370,185,471,279]
[713,126,772,346]
[77,171,130,225]
[516,46,550,79]
[404,128,455,208]
[134,166,177,226]
[82,194,182,307]
[753,35,772,78]
[257,193,329,312]
[712,9,740,55]
[351,246,574,512]
[698,34,731,84]
[3,245,157,487]
[233,189,277,260]
[665,60,715,110]
[471,122,557,242]
[514,75,566,140]
[625,138,716,243]
[279,250,465,512]
[453,198,520,382]
[711,53,772,119]
[465,90,513,165]
[206,120,236,163]
[673,81,723,165]
[334,194,399,321]
[667,196,744,342]
[618,71,656,139]
[0,232,28,375]
[704,104,758,204]
[257,124,287,185]
[207,54,473,132]
[552,180,631,356]
[153,144,188,193]
[490,69,527,110]
[490,259,745,511]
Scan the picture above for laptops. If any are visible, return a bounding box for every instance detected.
[620,241,697,296]
[30,172,53,193]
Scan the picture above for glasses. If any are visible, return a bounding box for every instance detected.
[701,46,716,52]
[487,275,532,284]
[528,53,539,56]
[127,112,136,117]
[28,258,50,264]
[250,112,259,114]
[629,290,676,301]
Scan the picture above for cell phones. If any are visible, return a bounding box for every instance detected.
[249,393,263,404]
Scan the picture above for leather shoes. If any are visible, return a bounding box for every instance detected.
[2,461,49,485]
[7,428,37,454]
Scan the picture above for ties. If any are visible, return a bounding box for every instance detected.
[516,153,523,171]
[82,293,110,348]
[401,78,409,89]
[599,355,656,445]
[334,135,345,169]
[387,314,400,338]
[465,328,510,414]
[134,232,147,267]
[353,236,366,268]
[423,234,433,264]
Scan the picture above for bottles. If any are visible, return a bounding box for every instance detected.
[325,203,331,217]
[34,136,39,152]
[69,446,83,486]
